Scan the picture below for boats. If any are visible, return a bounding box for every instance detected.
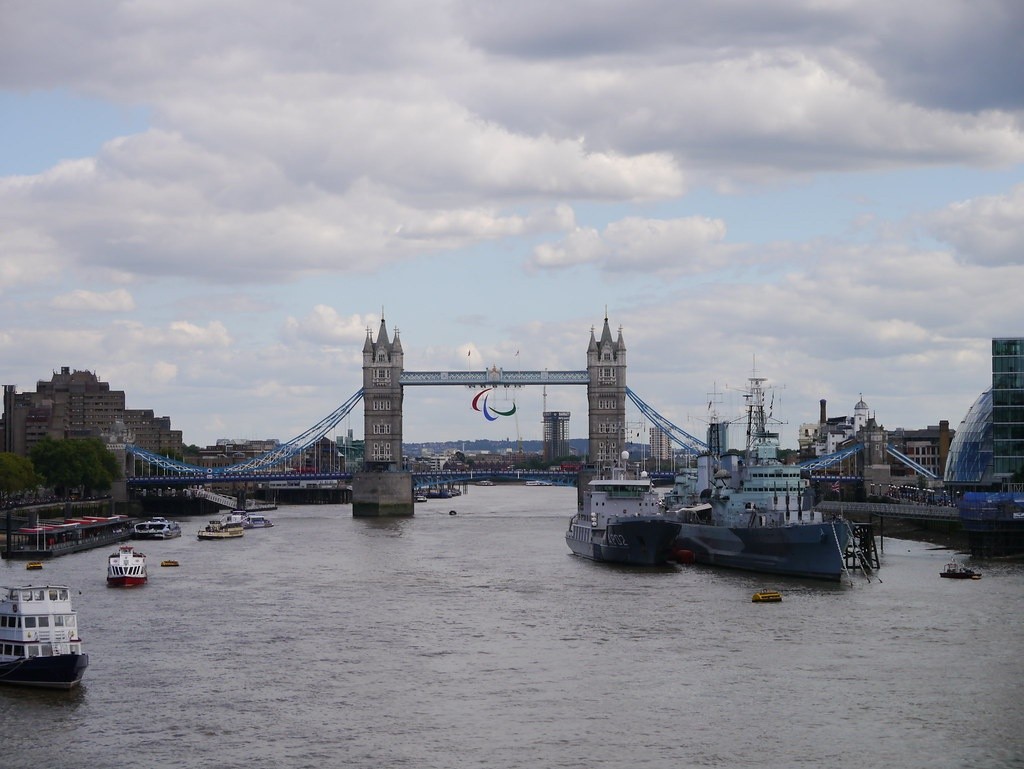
[525,480,553,487]
[663,353,852,585]
[222,510,274,529]
[563,420,683,568]
[134,517,182,541]
[0,584,90,691]
[197,520,244,539]
[106,546,148,588]
[414,489,462,502]
[939,558,983,580]
[475,481,494,486]
[12,513,139,559]
